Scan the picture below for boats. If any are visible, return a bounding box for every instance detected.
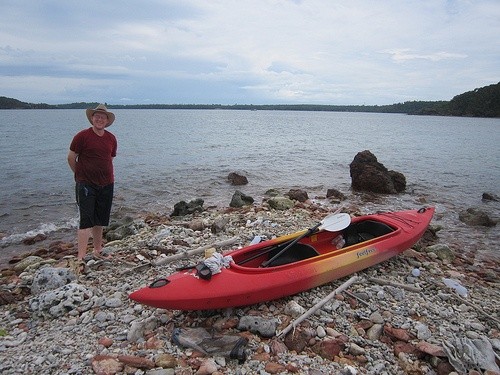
[128,205,435,310]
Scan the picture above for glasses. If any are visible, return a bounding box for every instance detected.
[93,114,110,122]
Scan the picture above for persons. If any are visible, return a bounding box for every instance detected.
[67,104,118,273]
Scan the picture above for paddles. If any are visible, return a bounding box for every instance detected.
[235,212,352,266]
[260,207,344,266]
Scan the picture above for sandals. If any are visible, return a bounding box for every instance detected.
[94,251,113,262]
[78,260,89,274]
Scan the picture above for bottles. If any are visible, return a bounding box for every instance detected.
[412,267,420,276]
[441,277,468,295]
[248,236,261,245]
[336,238,346,250]
[331,233,344,244]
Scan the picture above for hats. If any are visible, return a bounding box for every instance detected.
[85,103,115,128]
